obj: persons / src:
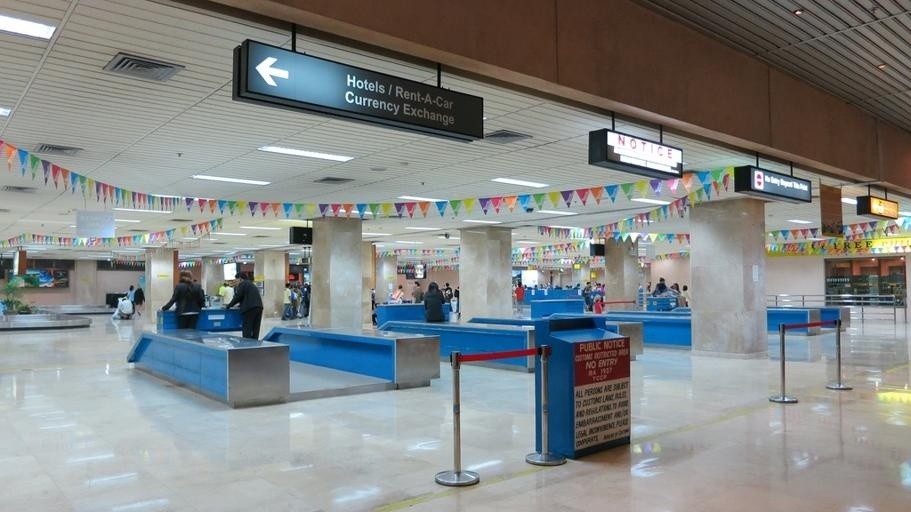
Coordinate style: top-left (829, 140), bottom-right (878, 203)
top-left (219, 270), bottom-right (263, 342)
top-left (513, 277), bottom-right (691, 313)
top-left (371, 279), bottom-right (460, 325)
top-left (280, 282), bottom-right (311, 320)
top-left (117, 283), bottom-right (146, 320)
top-left (157, 270), bottom-right (205, 330)
